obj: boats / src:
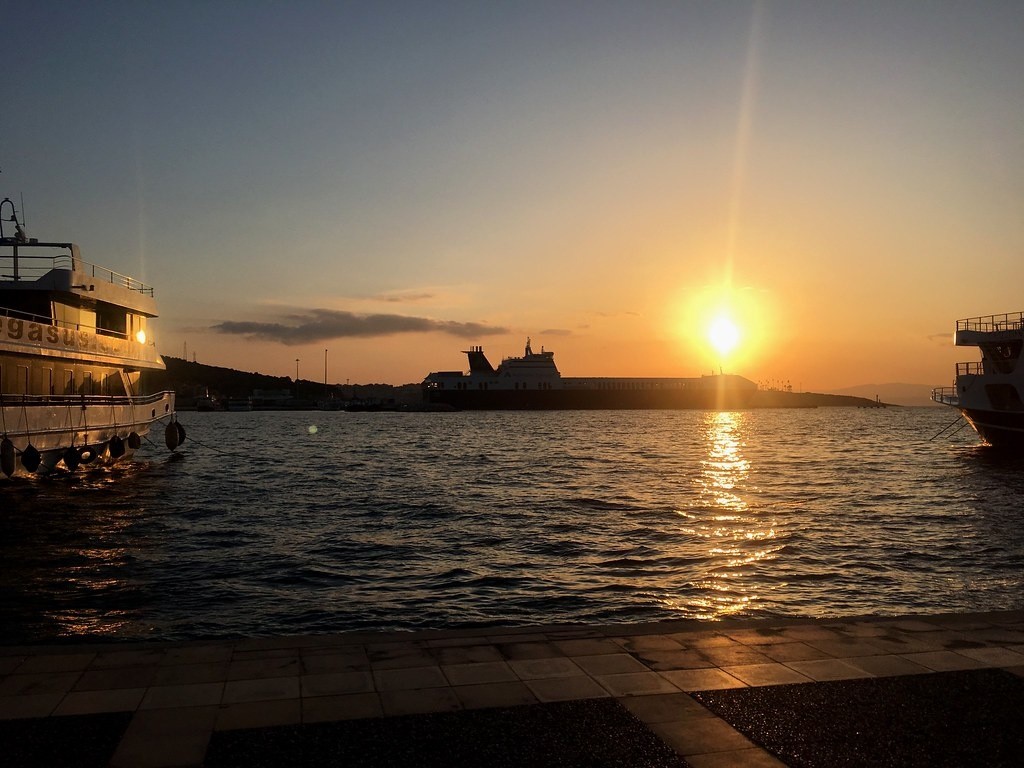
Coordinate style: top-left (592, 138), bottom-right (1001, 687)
top-left (194, 388), bottom-right (252, 412)
top-left (930, 311), bottom-right (1024, 458)
top-left (419, 337), bottom-right (757, 410)
top-left (0, 191), bottom-right (187, 479)
top-left (340, 396), bottom-right (415, 413)
